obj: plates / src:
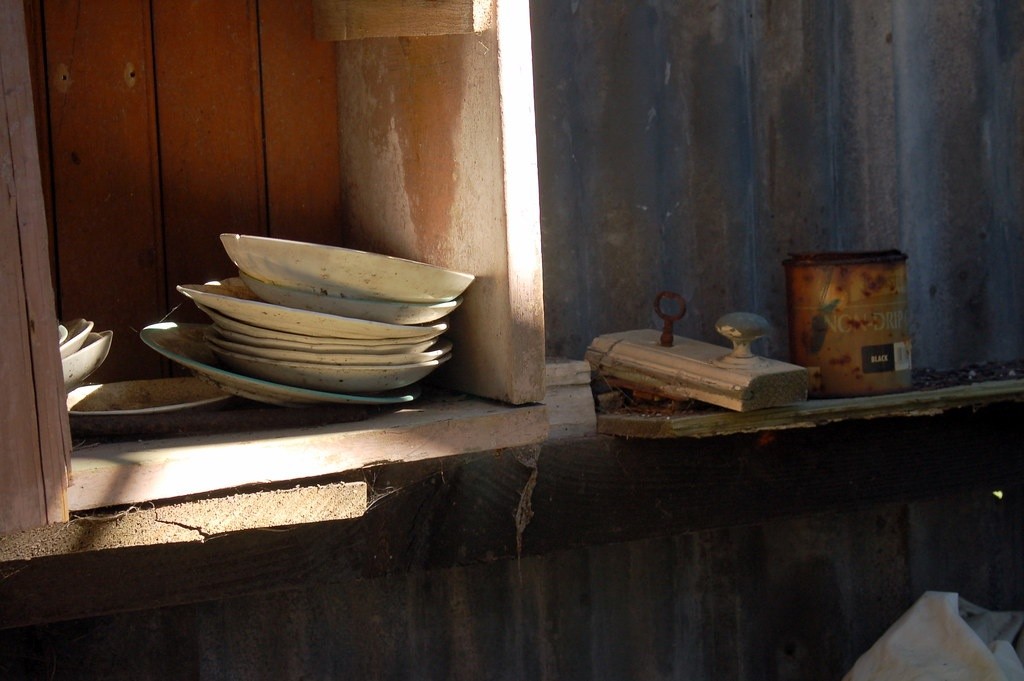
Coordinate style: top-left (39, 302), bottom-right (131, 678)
top-left (59, 233), bottom-right (474, 436)
